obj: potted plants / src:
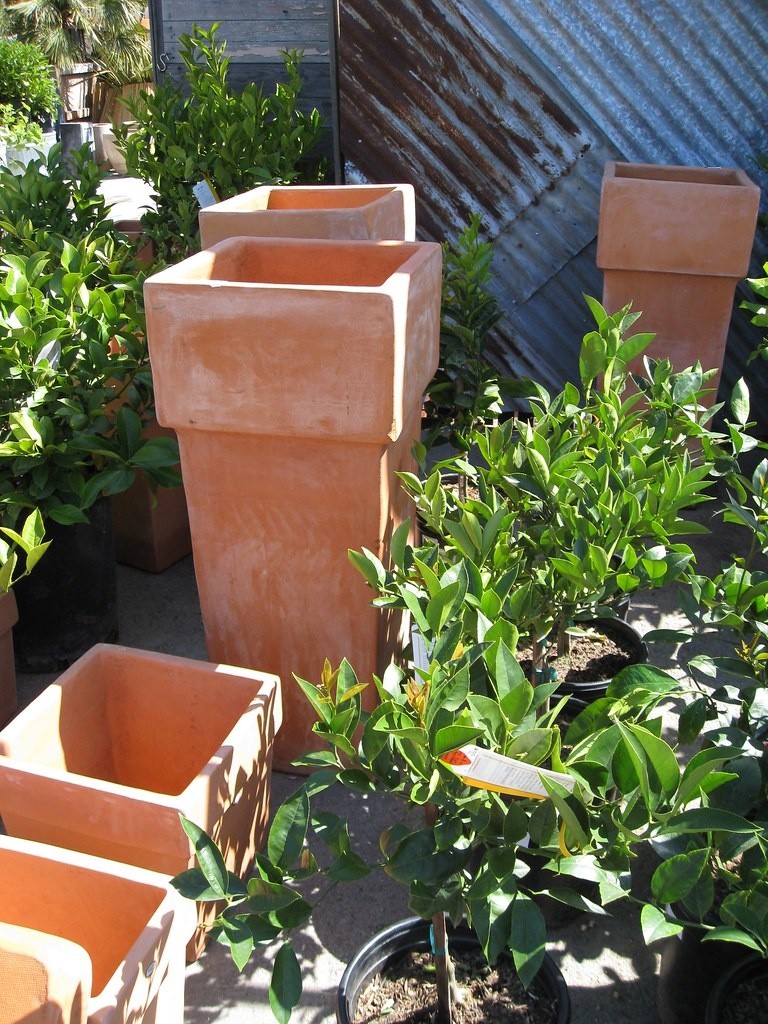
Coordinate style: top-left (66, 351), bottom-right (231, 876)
top-left (0, 0), bottom-right (764, 1022)
